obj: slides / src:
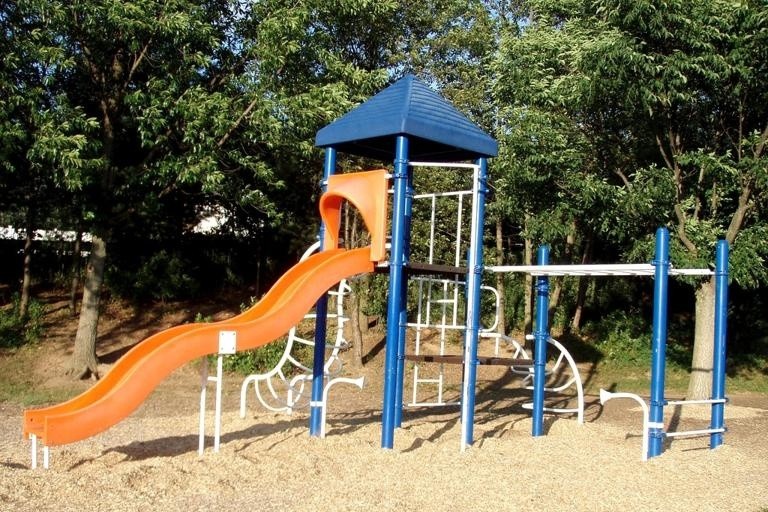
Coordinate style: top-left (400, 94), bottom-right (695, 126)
top-left (25, 238), bottom-right (374, 446)
